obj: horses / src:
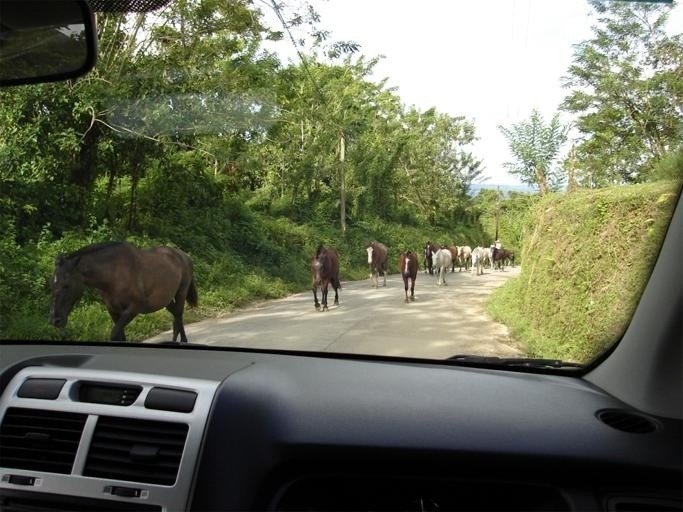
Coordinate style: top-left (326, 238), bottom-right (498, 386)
top-left (311, 245), bottom-right (341, 313)
top-left (431, 249), bottom-right (452, 287)
top-left (366, 240), bottom-right (389, 288)
top-left (399, 249), bottom-right (418, 302)
top-left (423, 240), bottom-right (514, 276)
top-left (47, 242), bottom-right (198, 341)
